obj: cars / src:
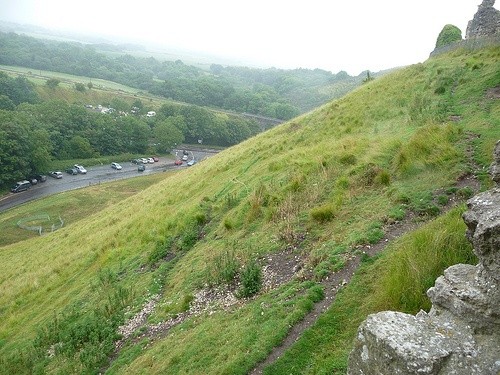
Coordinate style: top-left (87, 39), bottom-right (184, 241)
top-left (28, 178), bottom-right (37, 185)
top-left (175, 160), bottom-right (183, 165)
top-left (132, 155), bottom-right (158, 172)
top-left (111, 162), bottom-right (122, 170)
top-left (183, 150), bottom-right (189, 155)
top-left (187, 159), bottom-right (195, 166)
top-left (10, 180), bottom-right (32, 193)
top-left (182, 155), bottom-right (188, 161)
top-left (50, 171), bottom-right (63, 178)
top-left (86, 105), bottom-right (93, 108)
top-left (72, 165), bottom-right (87, 174)
top-left (65, 168), bottom-right (78, 176)
top-left (147, 111), bottom-right (156, 117)
top-left (98, 105), bottom-right (102, 109)
top-left (35, 174), bottom-right (47, 183)
